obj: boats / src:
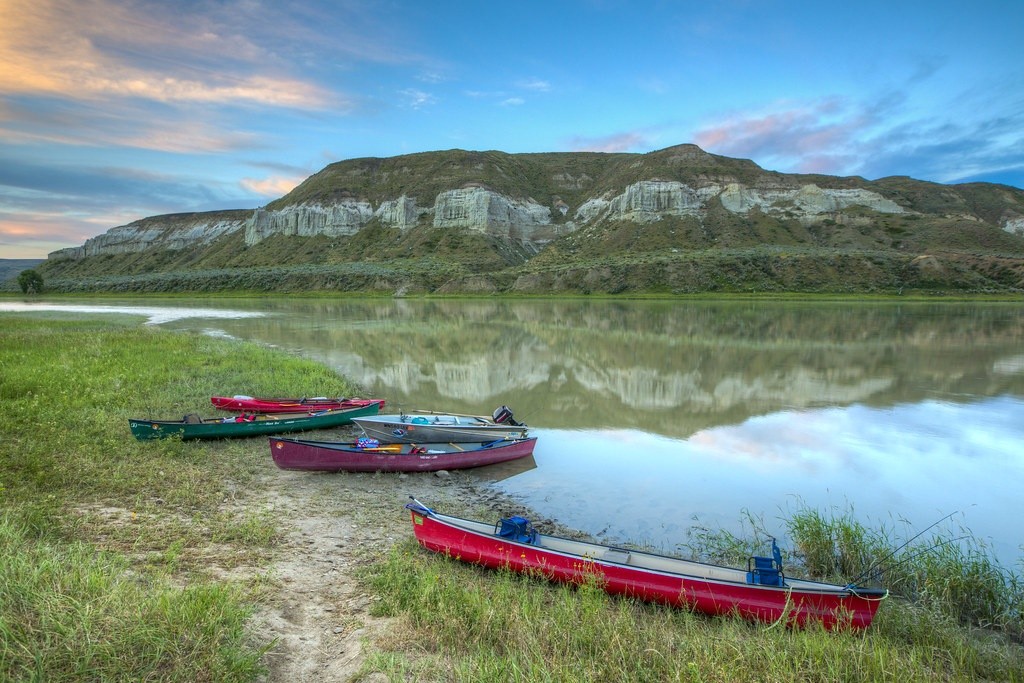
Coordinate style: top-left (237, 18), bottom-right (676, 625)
top-left (211, 396), bottom-right (384, 412)
top-left (269, 436), bottom-right (538, 472)
top-left (127, 401), bottom-right (380, 441)
top-left (350, 414), bottom-right (525, 444)
top-left (405, 502), bottom-right (891, 632)
